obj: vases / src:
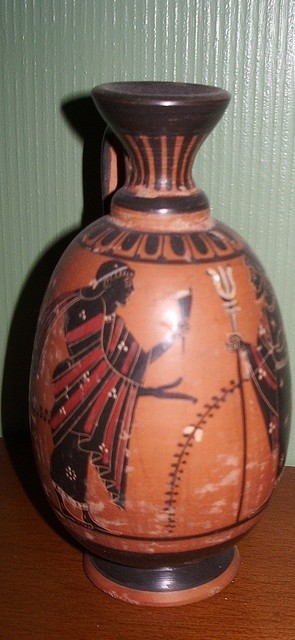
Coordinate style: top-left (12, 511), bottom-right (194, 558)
top-left (27, 80), bottom-right (293, 607)
